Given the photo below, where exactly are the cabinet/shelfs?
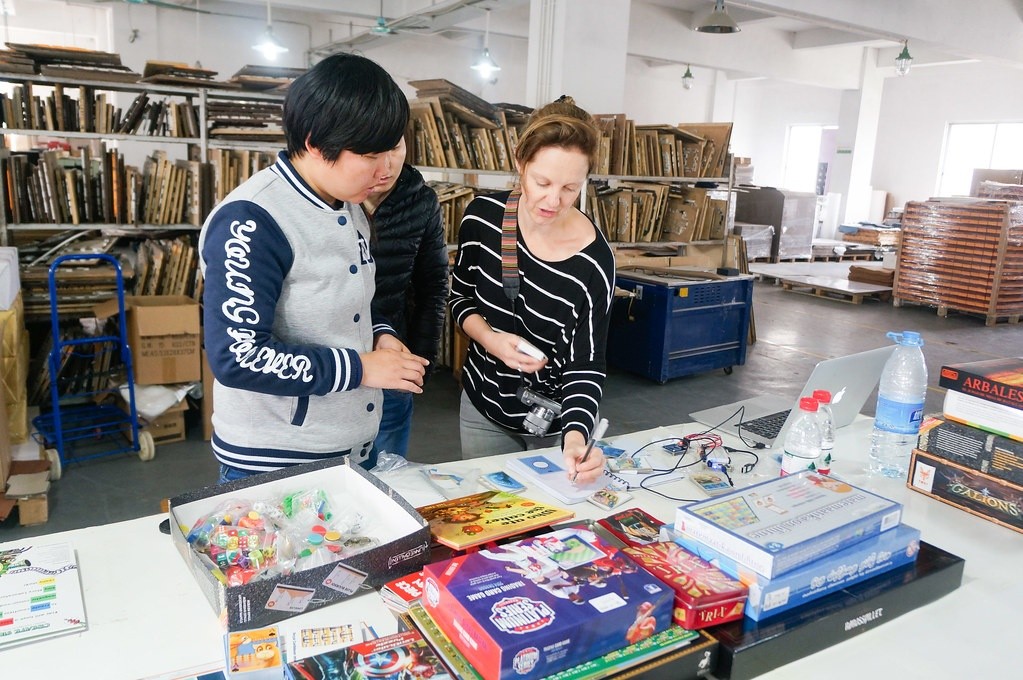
[0,74,735,421]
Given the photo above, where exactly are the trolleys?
[30,252,156,481]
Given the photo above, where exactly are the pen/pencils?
[573,419,611,484]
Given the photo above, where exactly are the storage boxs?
[195,328,216,442]
[166,455,430,631]
[677,469,903,580]
[943,390,1023,440]
[712,544,966,680]
[905,450,1023,533]
[92,294,205,386]
[917,417,1023,486]
[939,357,1023,408]
[660,524,920,622]
[117,396,189,447]
[423,527,676,680]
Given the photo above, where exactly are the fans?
[324,0,430,36]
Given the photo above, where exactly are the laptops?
[688,344,898,448]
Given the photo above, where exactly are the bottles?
[781,397,821,479]
[866,330,928,478]
[811,391,835,476]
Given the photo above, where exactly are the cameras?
[517,386,564,438]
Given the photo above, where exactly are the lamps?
[470,8,501,73]
[252,1,288,58]
[895,40,912,76]
[694,0,741,34]
[682,64,694,89]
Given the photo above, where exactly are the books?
[507,449,629,506]
[907,356,1023,534]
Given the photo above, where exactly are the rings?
[517,364,522,372]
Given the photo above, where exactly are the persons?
[359,131,449,468]
[197,52,430,485]
[446,95,616,484]
